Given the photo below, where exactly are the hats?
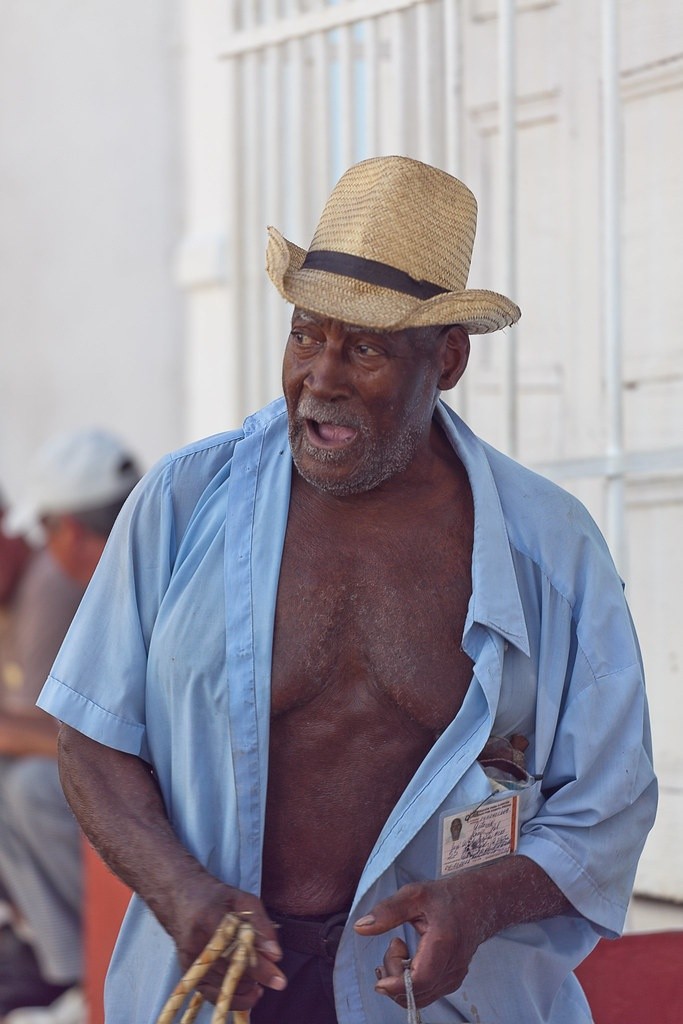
[264,156,522,335]
[2,424,138,537]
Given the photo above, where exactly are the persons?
[0,429,143,1024]
[36,156,659,1024]
[445,818,465,843]
